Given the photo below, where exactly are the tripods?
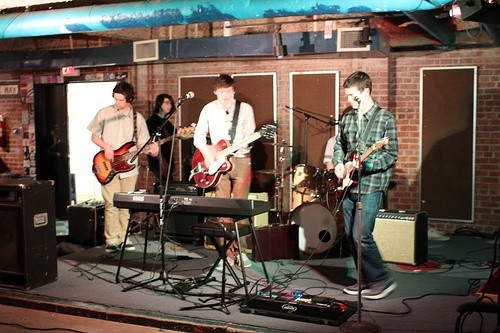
[181,219,251,314]
[123,98,186,300]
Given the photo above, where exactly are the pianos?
[112,191,273,302]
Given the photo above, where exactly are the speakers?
[371,207,428,266]
[0,178,59,290]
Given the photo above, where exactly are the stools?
[192,223,254,294]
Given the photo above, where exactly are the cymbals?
[256,169,293,174]
[263,142,296,147]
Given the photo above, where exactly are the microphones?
[177,91,194,100]
[322,118,335,129]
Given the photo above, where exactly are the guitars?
[93,123,196,185]
[335,137,390,200]
[192,124,277,189]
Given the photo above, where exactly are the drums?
[290,198,347,255]
[321,169,342,200]
[292,164,319,196]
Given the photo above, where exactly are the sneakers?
[343,278,370,295]
[360,277,398,299]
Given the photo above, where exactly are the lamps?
[460,0,500,22]
[270,24,288,58]
[353,15,380,46]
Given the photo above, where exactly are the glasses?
[163,101,172,105]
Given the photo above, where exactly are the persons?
[323,70,398,300]
[88,82,158,254]
[193,74,257,270]
[147,94,194,228]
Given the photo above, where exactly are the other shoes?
[105,243,118,253]
[226,257,234,266]
[119,240,136,251]
[236,253,252,267]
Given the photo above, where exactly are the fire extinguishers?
[0,113,7,148]
